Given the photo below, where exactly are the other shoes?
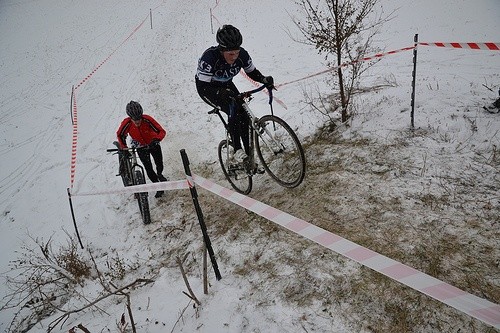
[155,191,164,198]
[159,176,167,182]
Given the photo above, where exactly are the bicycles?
[107,141,151,224]
[208,82,307,195]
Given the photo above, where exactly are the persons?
[117,101,167,198]
[194,24,274,174]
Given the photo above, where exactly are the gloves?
[224,89,235,102]
[153,139,160,146]
[264,76,274,87]
[124,152,133,159]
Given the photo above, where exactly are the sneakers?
[233,150,250,163]
[256,163,265,174]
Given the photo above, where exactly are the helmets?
[126,101,143,120]
[216,24,243,51]
